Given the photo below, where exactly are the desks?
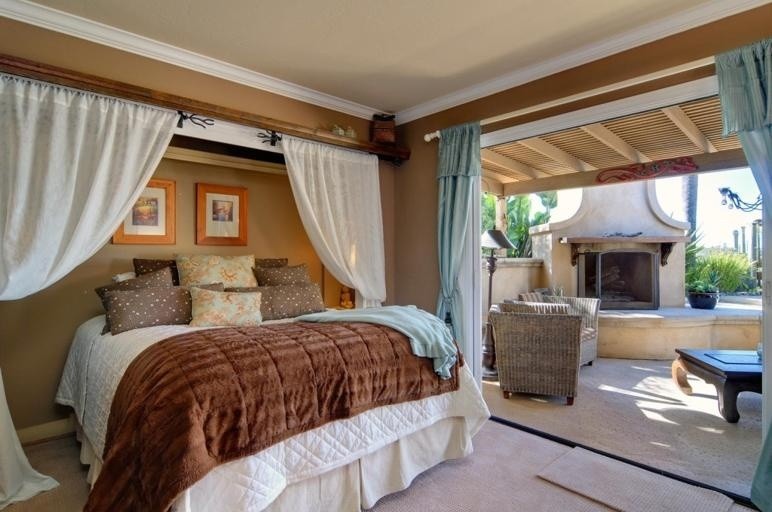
[671,347,763,424]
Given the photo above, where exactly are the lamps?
[479,229,519,383]
[718,185,762,213]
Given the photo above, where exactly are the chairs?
[517,292,601,369]
[488,301,583,408]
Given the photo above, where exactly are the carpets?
[537,442,733,512]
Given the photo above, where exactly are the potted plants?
[685,267,721,311]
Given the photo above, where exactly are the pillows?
[92,256,328,336]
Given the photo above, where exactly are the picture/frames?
[111,178,178,247]
[194,181,249,248]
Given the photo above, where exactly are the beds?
[57,255,491,511]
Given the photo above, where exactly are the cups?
[756,342,762,358]
